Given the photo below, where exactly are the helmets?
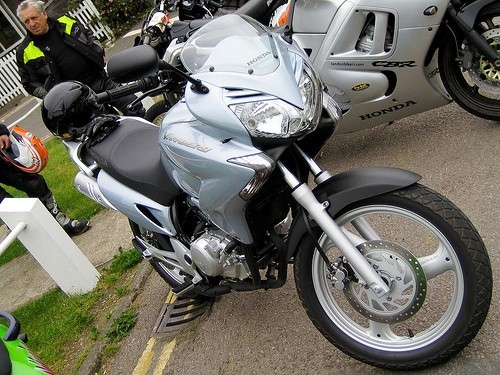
[0,126,48,174]
[41,81,100,140]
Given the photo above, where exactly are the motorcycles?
[131,0,500,127]
[41,0,496,372]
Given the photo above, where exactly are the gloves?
[81,114,119,148]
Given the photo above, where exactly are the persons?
[15,0,147,120]
[0,123,90,238]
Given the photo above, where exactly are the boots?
[40,193,90,237]
